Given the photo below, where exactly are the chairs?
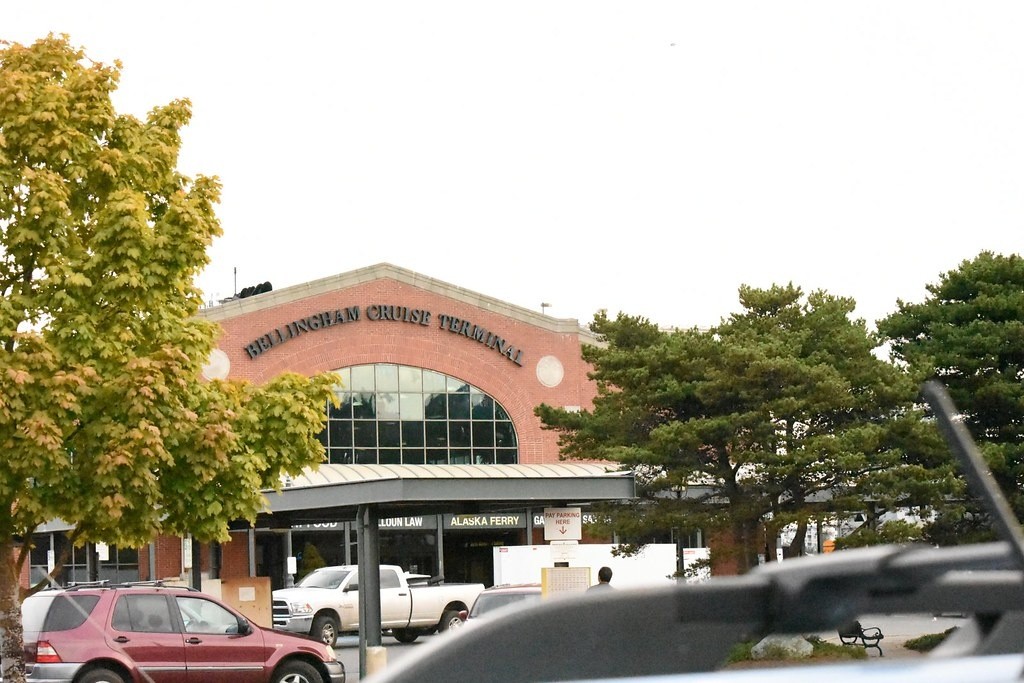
[130,610,168,633]
[837,621,883,657]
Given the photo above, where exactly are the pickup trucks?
[270,564,487,650]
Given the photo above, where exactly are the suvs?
[20,582,346,683]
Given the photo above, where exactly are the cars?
[465,585,545,625]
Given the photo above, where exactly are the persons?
[585,567,617,596]
